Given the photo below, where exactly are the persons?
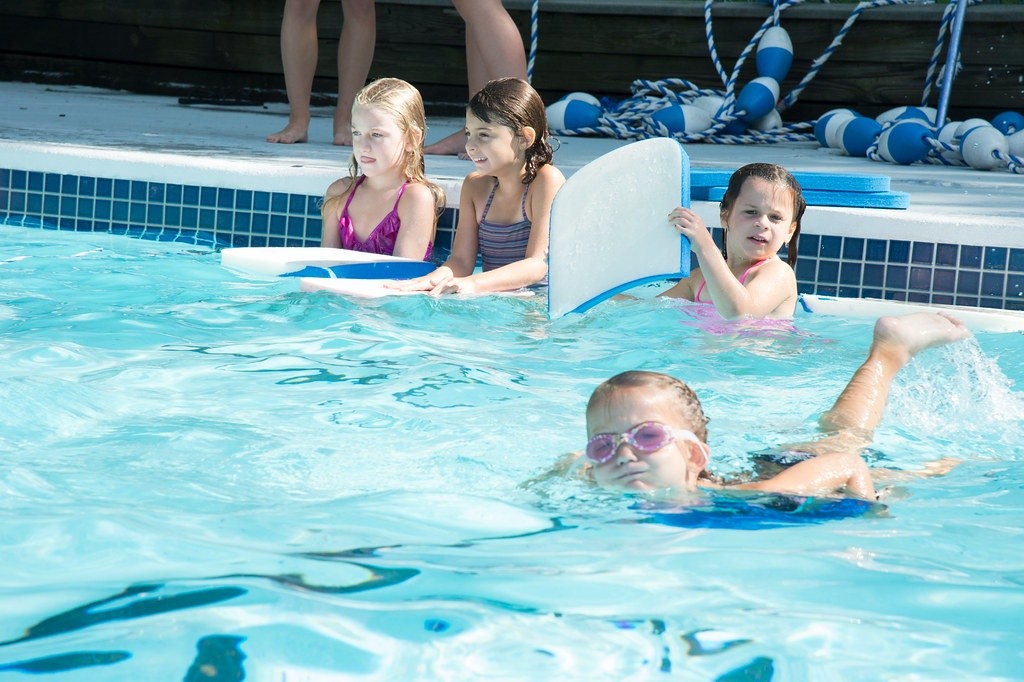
[610,161,806,323]
[265,1,380,146]
[517,310,973,504]
[319,77,438,262]
[416,1,529,163]
[381,77,567,300]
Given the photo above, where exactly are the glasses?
[584,421,675,464]
